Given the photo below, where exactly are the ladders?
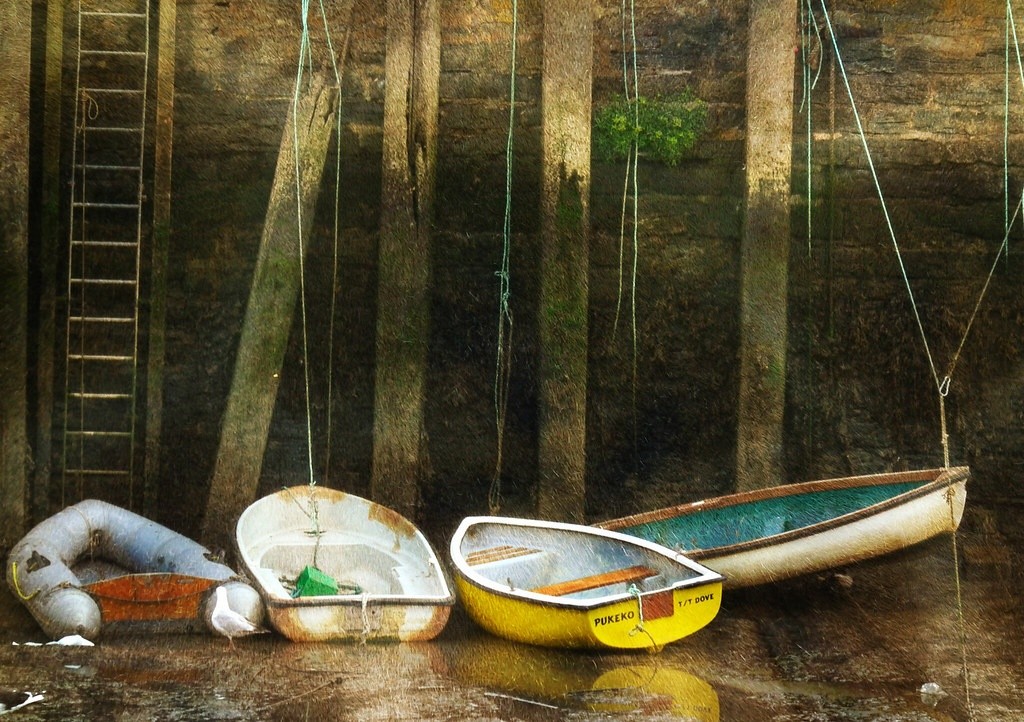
[59,2,152,509]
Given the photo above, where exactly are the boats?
[447,515,729,655]
[7,497,264,645]
[232,485,457,644]
[582,465,970,592]
